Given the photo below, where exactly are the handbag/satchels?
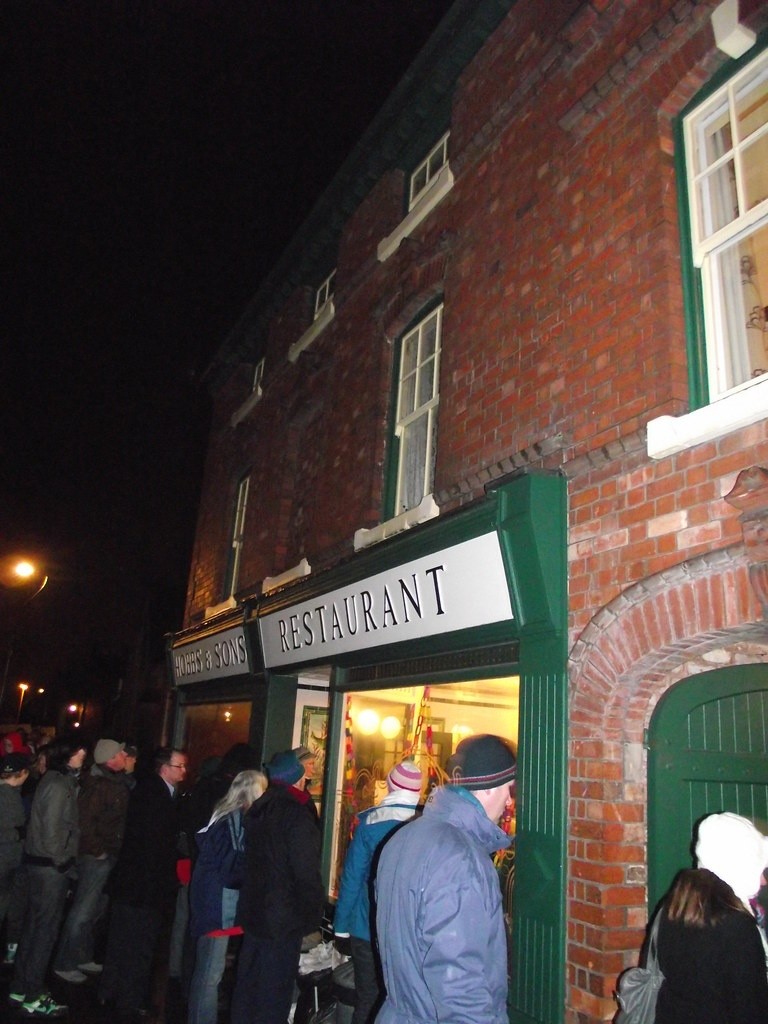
[613,908,664,1024]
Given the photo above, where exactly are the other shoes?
[55,971,87,983]
[77,959,103,972]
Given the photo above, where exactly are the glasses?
[167,763,185,768]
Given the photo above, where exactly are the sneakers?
[8,991,50,1006]
[19,993,67,1018]
[4,944,18,963]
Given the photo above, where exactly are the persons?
[639,810,768,1024]
[1,726,140,1018]
[114,748,194,1021]
[172,742,325,1024]
[333,735,519,1024]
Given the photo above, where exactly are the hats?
[444,734,516,790]
[266,750,305,785]
[695,810,768,904]
[387,762,422,793]
[294,747,315,762]
[0,752,33,773]
[94,739,125,763]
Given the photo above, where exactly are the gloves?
[334,936,352,957]
